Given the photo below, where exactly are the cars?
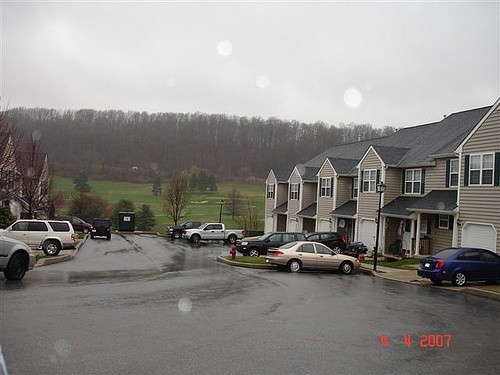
[265,242,361,275]
[0,235,35,280]
[416,248,500,287]
[236,231,348,257]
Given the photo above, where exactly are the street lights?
[218,200,224,223]
[373,182,388,269]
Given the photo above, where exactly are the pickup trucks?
[182,223,244,245]
[167,221,221,239]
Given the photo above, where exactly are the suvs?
[91,218,111,240]
[50,217,92,234]
[0,219,77,256]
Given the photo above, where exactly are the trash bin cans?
[118,211,136,232]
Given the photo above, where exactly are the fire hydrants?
[230,246,237,260]
